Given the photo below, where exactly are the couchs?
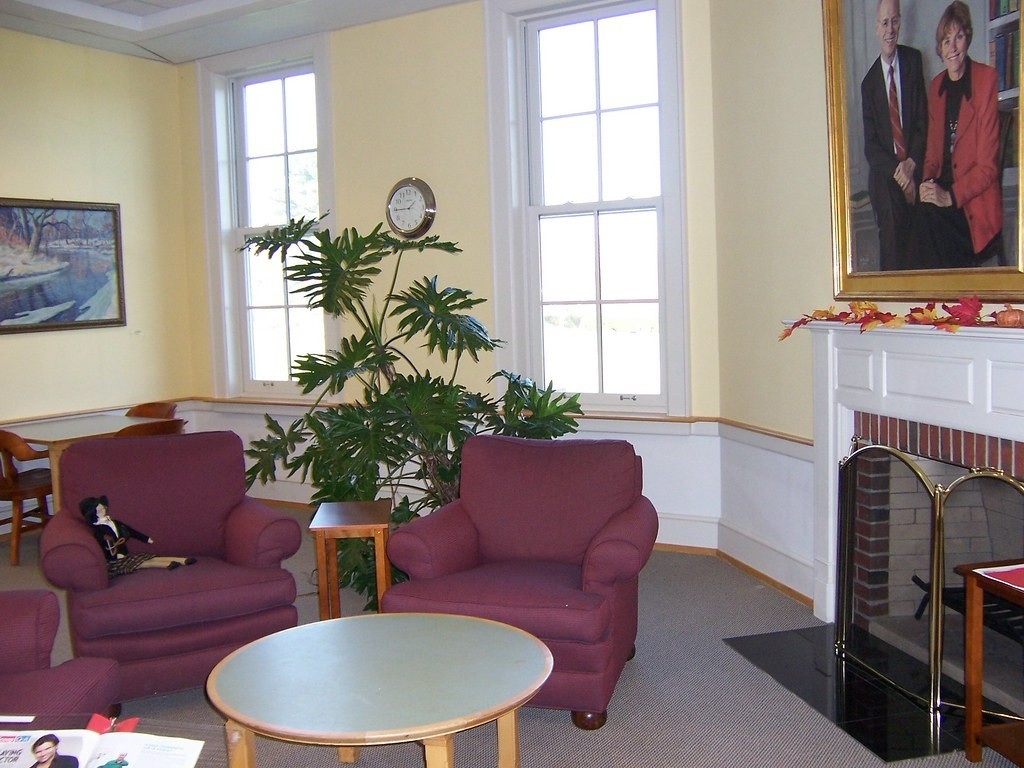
[381,434,659,729]
[40,428,302,718]
[0,589,123,729]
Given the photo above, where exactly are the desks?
[0,415,155,514]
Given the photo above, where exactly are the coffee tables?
[206,611,554,768]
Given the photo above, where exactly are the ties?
[888,66,906,160]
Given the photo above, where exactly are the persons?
[79,495,198,576]
[97,752,128,768]
[29,734,79,768]
[907,0,1002,269]
[860,0,926,272]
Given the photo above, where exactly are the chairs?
[0,429,53,566]
[125,401,177,419]
[113,419,184,436]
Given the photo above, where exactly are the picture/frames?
[821,0,1024,303]
[0,197,127,335]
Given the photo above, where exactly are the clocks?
[386,177,437,239]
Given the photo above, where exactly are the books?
[989,29,1019,92]
[998,107,1018,167]
[989,0,1021,21]
[0,730,205,768]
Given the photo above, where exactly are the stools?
[309,499,391,622]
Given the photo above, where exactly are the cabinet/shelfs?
[953,557,1024,768]
[984,0,1019,187]
